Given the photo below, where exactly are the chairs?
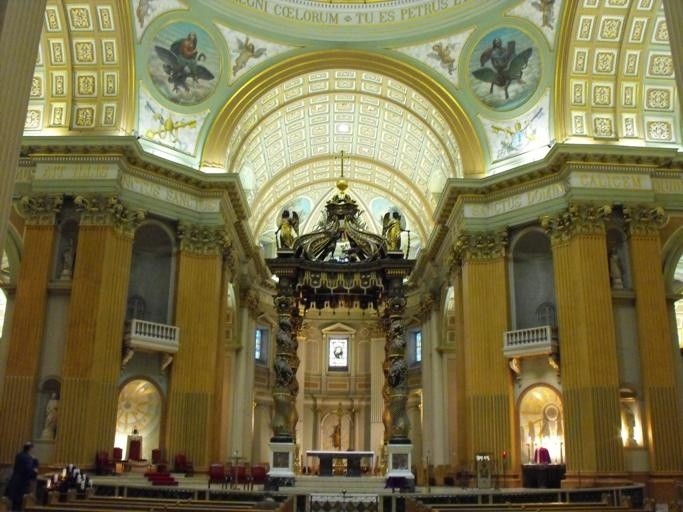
[96,433,269,489]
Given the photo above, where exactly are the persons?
[39,391,59,438]
[56,236,76,283]
[177,31,200,87]
[0,440,92,512]
[382,211,409,251]
[489,37,524,84]
[230,43,266,78]
[608,244,624,291]
[431,44,456,76]
[274,210,295,250]
